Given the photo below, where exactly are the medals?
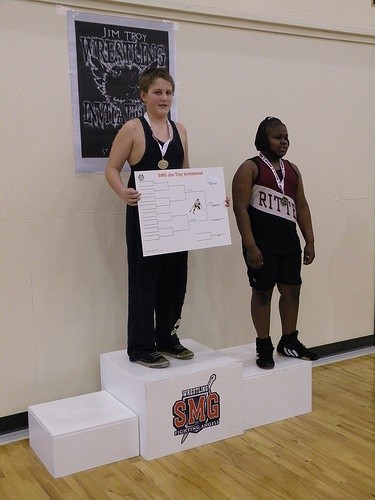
[158,160,168,170]
[281,197,288,206]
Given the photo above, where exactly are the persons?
[106,68,230,369]
[232,116,319,369]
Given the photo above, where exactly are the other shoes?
[156,342,194,360]
[133,351,170,369]
[276,331,319,361]
[255,336,275,370]
